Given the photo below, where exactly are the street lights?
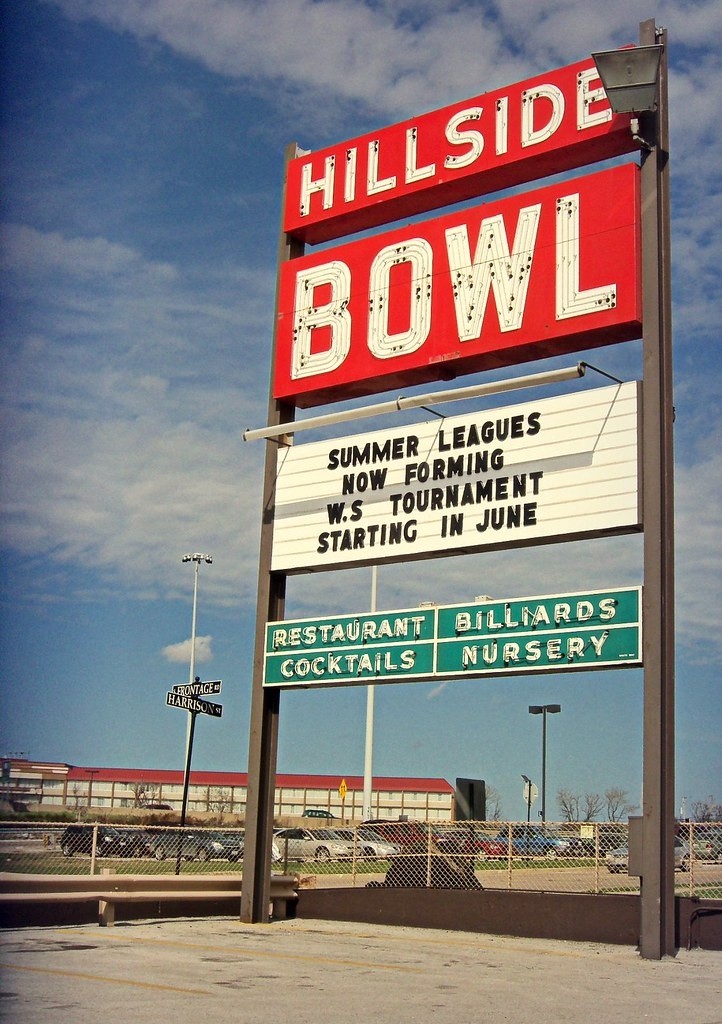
[181,552,212,819]
[529,704,561,831]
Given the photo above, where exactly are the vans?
[605,834,693,874]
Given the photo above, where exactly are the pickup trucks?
[496,825,593,861]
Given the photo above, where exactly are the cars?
[435,828,514,862]
[690,831,722,862]
[273,828,363,864]
[114,829,152,858]
[328,829,403,860]
[356,819,392,841]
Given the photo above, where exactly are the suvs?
[144,822,245,862]
[302,810,341,819]
[372,819,440,848]
[59,823,134,857]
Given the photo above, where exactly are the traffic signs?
[165,680,222,717]
